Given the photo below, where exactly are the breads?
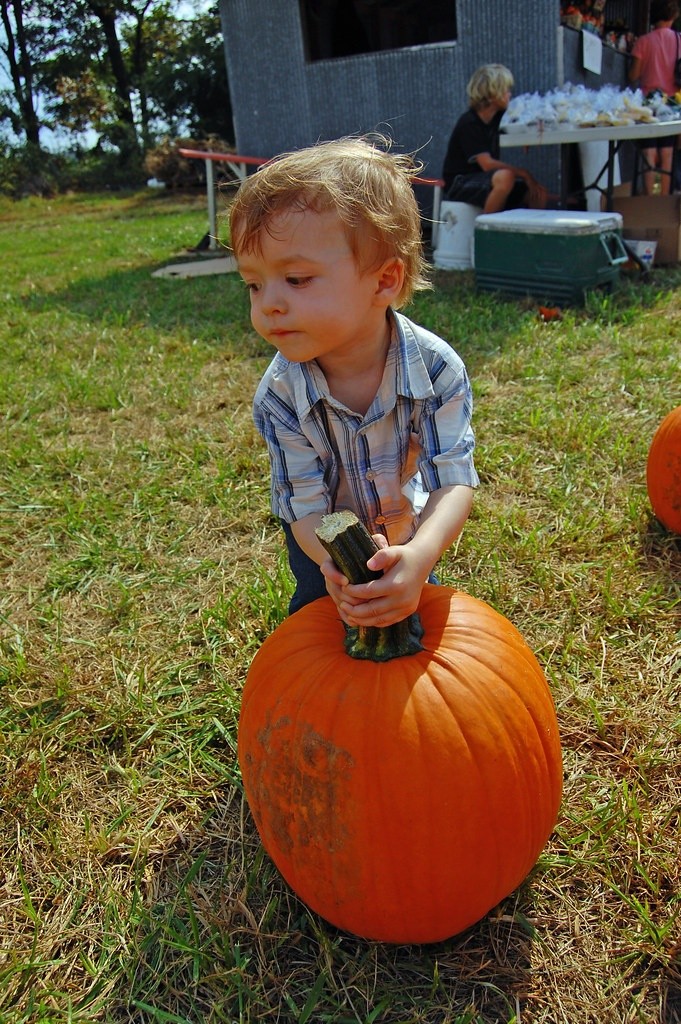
[573,105,654,126]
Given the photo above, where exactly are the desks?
[495,121,681,210]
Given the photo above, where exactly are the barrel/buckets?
[432,201,484,270]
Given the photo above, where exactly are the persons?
[627,0,681,195]
[442,63,549,214]
[226,136,481,629]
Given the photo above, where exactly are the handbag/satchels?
[674,31,681,88]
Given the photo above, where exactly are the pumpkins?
[647,404,681,538]
[238,510,563,945]
[538,305,558,321]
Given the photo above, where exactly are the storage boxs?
[599,182,681,268]
[476,207,629,312]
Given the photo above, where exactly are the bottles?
[619,35,626,51]
